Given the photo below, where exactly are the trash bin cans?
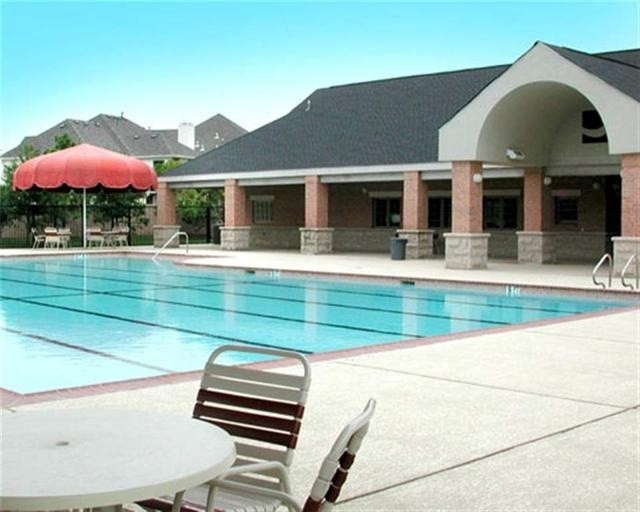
[213,219,224,244]
[390,236,407,260]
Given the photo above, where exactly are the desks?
[0,404,236,512]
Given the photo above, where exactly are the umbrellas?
[12,143,158,248]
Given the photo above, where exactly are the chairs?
[115,344,377,510]
[31,223,131,250]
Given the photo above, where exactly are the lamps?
[506,148,525,161]
